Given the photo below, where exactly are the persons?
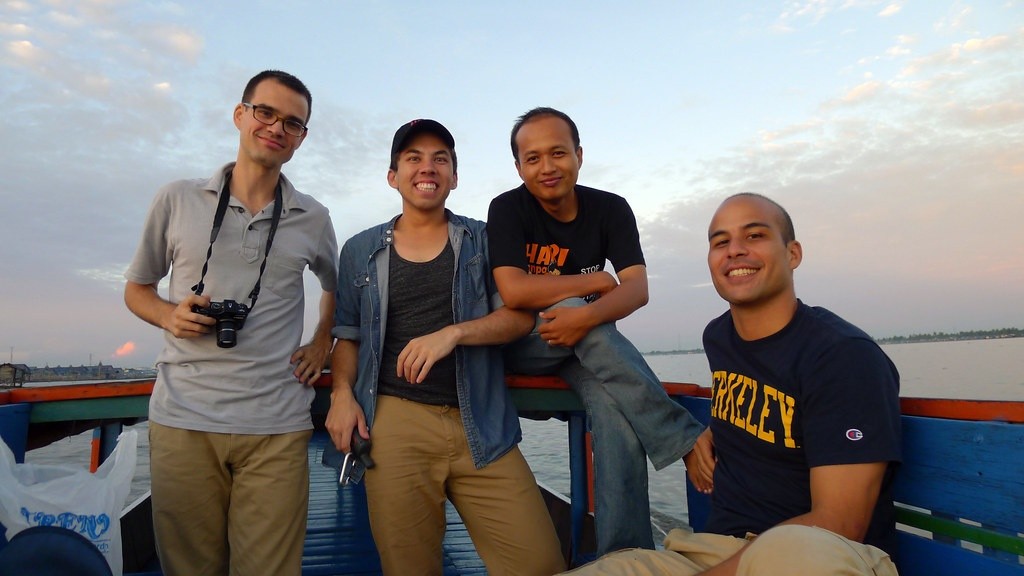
[326,118,568,575]
[125,71,339,576]
[486,108,712,560]
[555,194,898,575]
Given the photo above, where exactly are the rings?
[549,340,552,346]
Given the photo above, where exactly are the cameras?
[190,300,250,349]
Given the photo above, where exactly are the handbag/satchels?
[0,431,138,576]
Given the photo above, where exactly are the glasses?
[243,103,308,137]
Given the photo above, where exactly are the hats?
[392,118,455,154]
